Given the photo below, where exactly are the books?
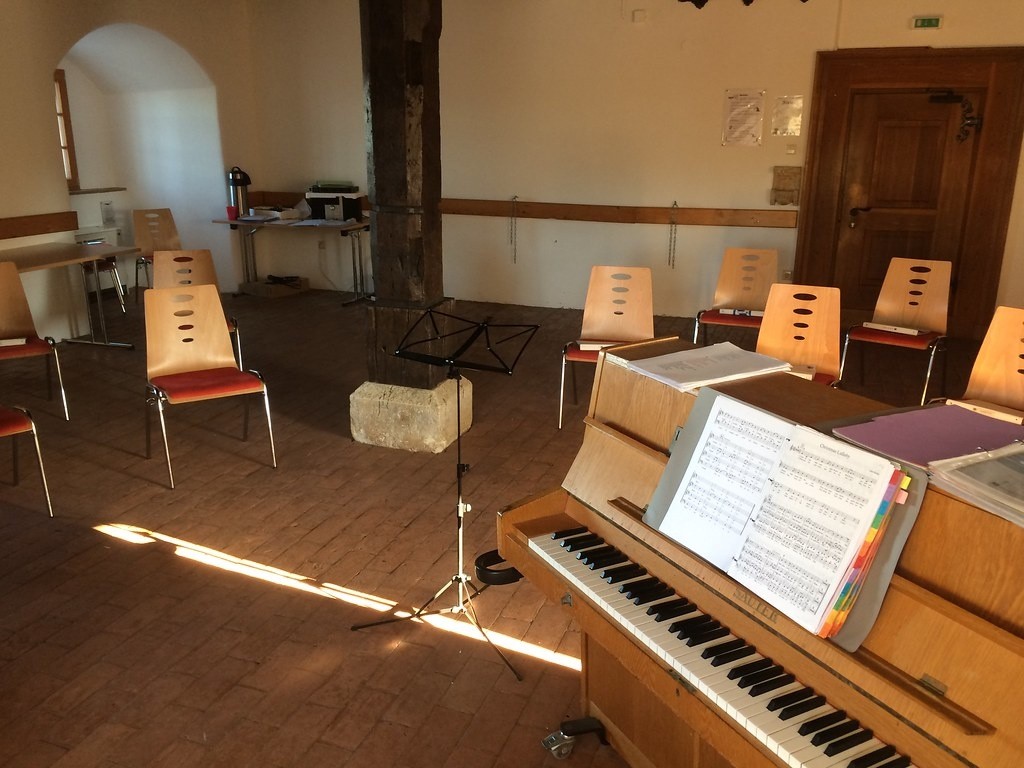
[641,386,928,653]
[831,404,1024,528]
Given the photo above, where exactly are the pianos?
[494,332,1024,768]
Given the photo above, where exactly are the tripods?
[352,304,540,680]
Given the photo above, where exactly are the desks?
[213,217,372,304]
[0,242,141,349]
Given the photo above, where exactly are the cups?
[225,206,235,222]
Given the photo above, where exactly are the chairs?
[692,249,777,348]
[927,306,1024,422]
[0,404,53,518]
[133,207,183,302]
[839,255,952,407]
[558,264,654,431]
[142,285,277,490]
[154,250,244,373]
[755,284,842,390]
[0,260,71,422]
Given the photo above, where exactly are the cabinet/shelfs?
[76,225,130,293]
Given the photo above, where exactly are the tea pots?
[230,166,252,217]
